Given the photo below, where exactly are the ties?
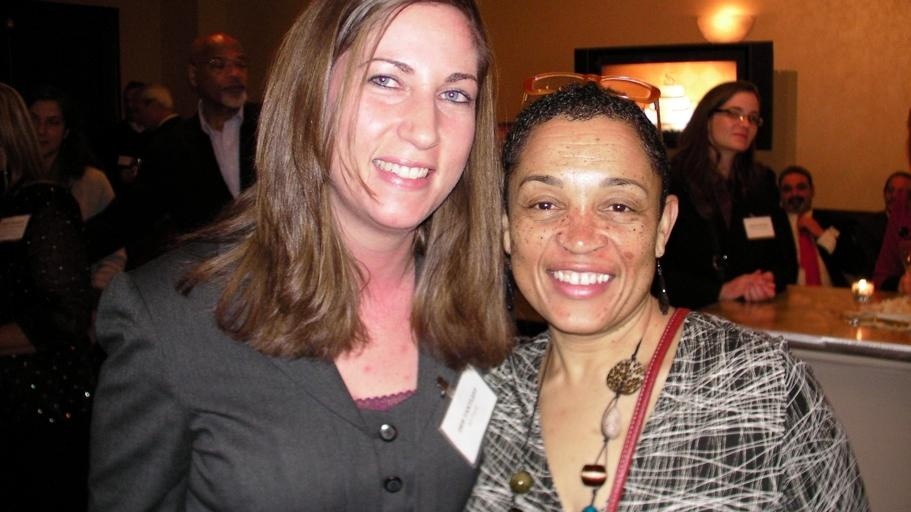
[796,213,823,285]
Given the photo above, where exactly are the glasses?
[515,70,665,147]
[716,107,765,128]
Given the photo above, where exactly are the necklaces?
[510,340,649,511]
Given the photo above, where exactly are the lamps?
[691,13,753,43]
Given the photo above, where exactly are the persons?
[1,27,264,512]
[650,79,801,311]
[857,170,911,285]
[83,2,486,511]
[773,164,857,289]
[457,77,873,511]
[871,187,911,296]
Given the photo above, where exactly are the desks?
[693,284,911,511]
